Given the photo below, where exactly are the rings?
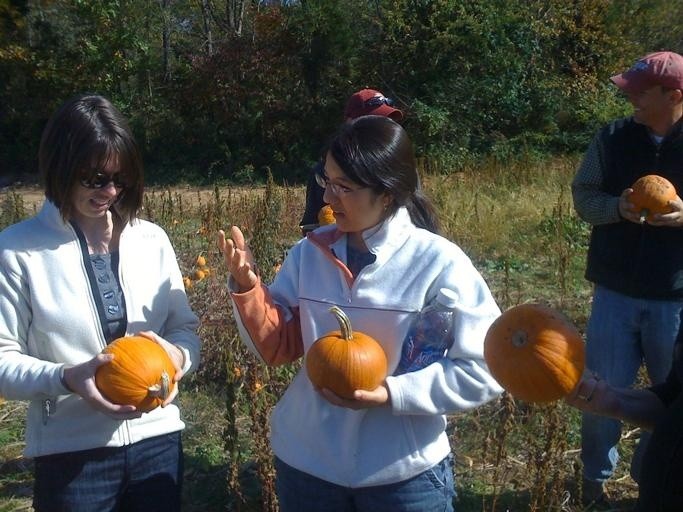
[576,393,591,402]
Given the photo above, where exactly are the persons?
[569,52,682,509]
[0,93,203,512]
[215,113,507,512]
[563,310,683,511]
[299,87,420,238]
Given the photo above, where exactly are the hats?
[343,88,402,123]
[609,51,683,94]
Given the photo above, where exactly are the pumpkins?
[183,255,210,287]
[305,306,388,400]
[95,335,176,412]
[318,204,336,226]
[627,174,676,225]
[483,304,584,402]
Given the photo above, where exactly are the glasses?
[313,169,369,196]
[364,95,393,110]
[629,57,649,78]
[77,166,135,190]
